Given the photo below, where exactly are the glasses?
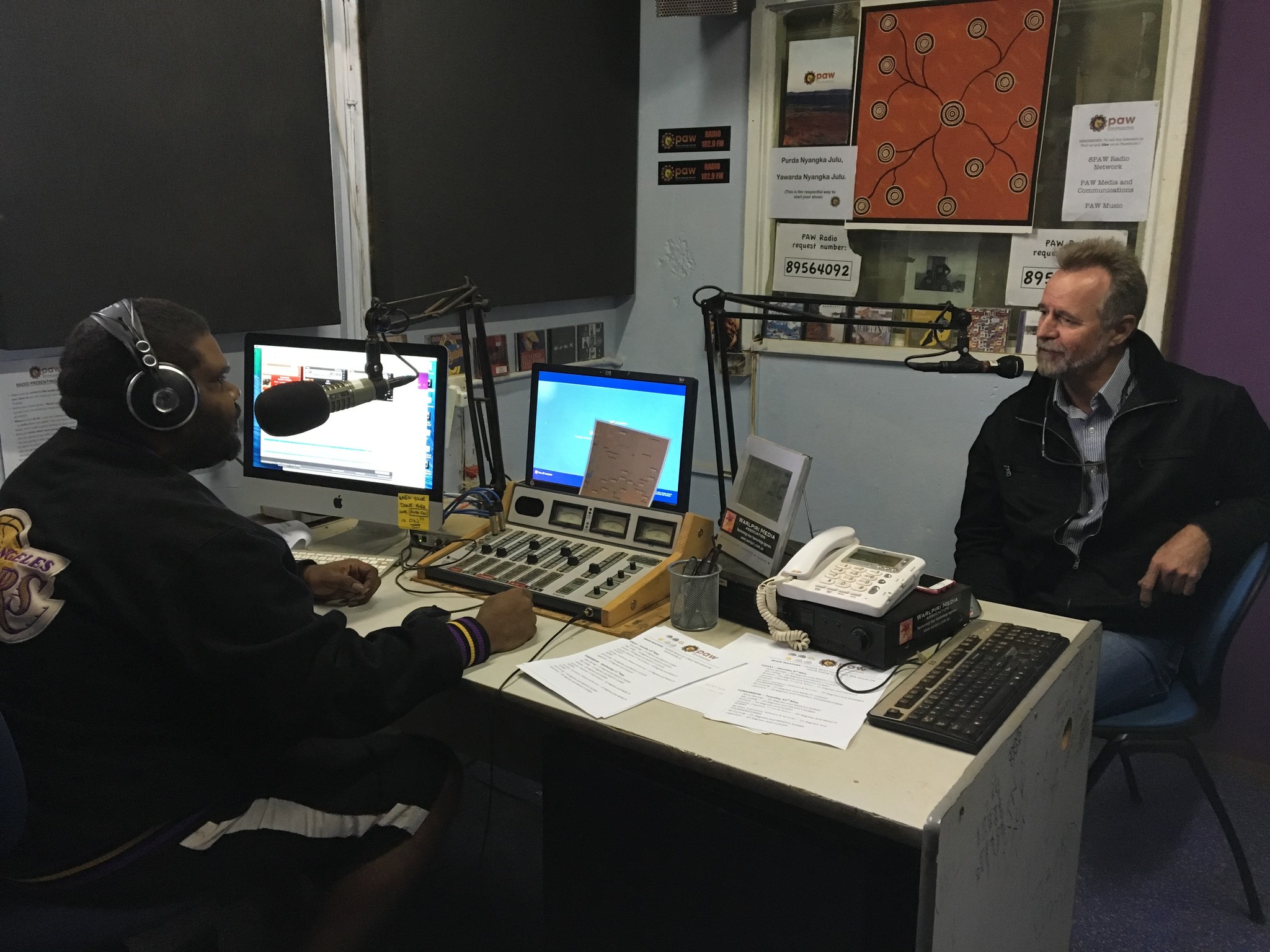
[1042,395,1124,466]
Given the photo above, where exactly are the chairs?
[1084,541,1270,926]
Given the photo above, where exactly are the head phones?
[90,298,198,433]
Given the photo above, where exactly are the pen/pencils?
[701,544,722,589]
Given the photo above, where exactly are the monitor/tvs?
[243,333,451,559]
[524,362,700,515]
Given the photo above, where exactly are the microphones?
[917,354,1023,379]
[254,376,418,438]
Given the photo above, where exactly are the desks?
[249,511,1102,952]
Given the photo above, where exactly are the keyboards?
[290,548,400,578]
[866,620,1071,758]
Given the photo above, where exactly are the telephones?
[775,526,926,617]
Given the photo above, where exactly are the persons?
[0,297,536,952]
[953,237,1270,721]
